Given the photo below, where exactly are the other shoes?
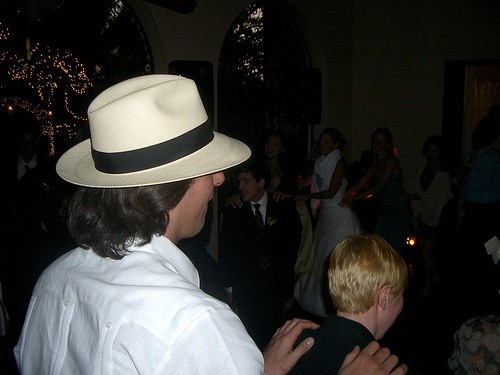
[280,297,297,317]
[418,287,430,303]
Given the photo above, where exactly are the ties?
[253,204,264,228]
[24,165,36,176]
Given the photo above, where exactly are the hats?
[56,74,252,189]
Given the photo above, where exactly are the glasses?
[20,140,34,145]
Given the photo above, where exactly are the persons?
[337,114,500,285]
[286,233,409,375]
[12,73,410,374]
[291,126,365,319]
[219,129,300,322]
